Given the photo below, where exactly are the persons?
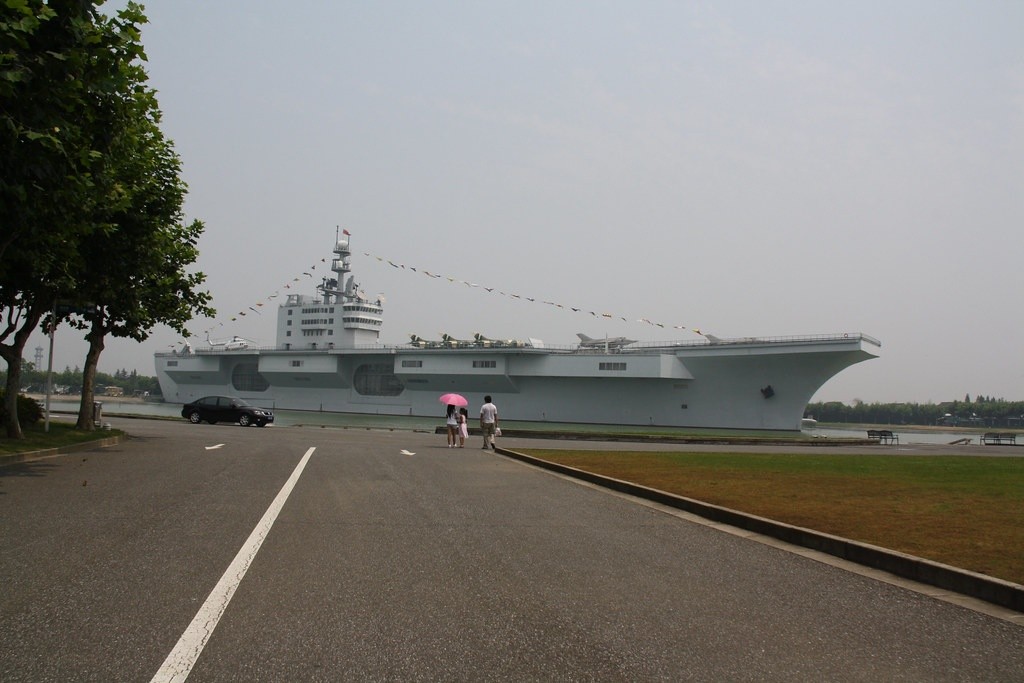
[480,395,499,450]
[445,403,460,448]
[457,408,469,448]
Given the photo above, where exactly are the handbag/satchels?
[494,426,502,437]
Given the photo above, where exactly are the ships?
[154,223,882,433]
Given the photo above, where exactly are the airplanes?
[576,332,637,348]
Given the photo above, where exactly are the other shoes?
[453,444,457,448]
[491,443,495,449]
[459,444,463,448]
[448,444,451,447]
[482,446,488,449]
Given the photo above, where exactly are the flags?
[343,228,349,235]
[169,259,327,348]
[364,252,704,336]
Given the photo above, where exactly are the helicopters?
[206,331,260,350]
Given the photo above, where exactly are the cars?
[181,395,274,427]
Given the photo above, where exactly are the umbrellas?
[439,393,468,406]
